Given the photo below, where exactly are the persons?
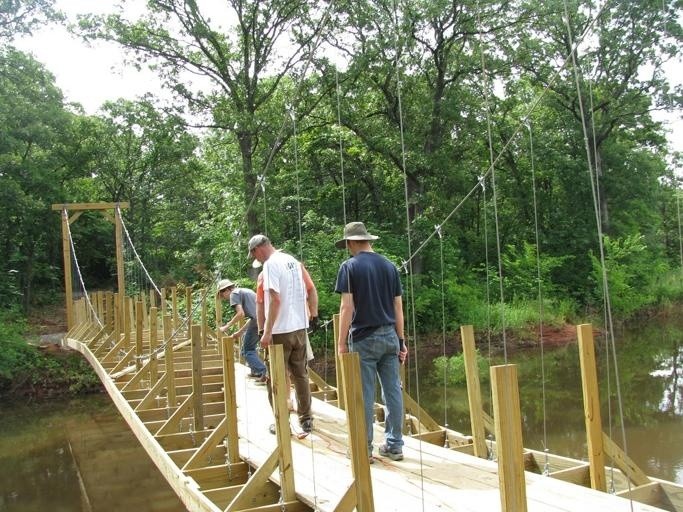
[334,221,408,461]
[252,248,314,411]
[217,279,268,386]
[247,235,318,437]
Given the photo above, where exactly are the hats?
[335,222,378,249]
[247,235,268,259]
[217,279,235,300]
[252,249,284,268]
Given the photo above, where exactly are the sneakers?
[346,448,374,464]
[270,424,293,436]
[299,420,313,431]
[248,374,269,384]
[379,444,403,461]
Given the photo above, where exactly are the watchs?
[258,329,264,334]
